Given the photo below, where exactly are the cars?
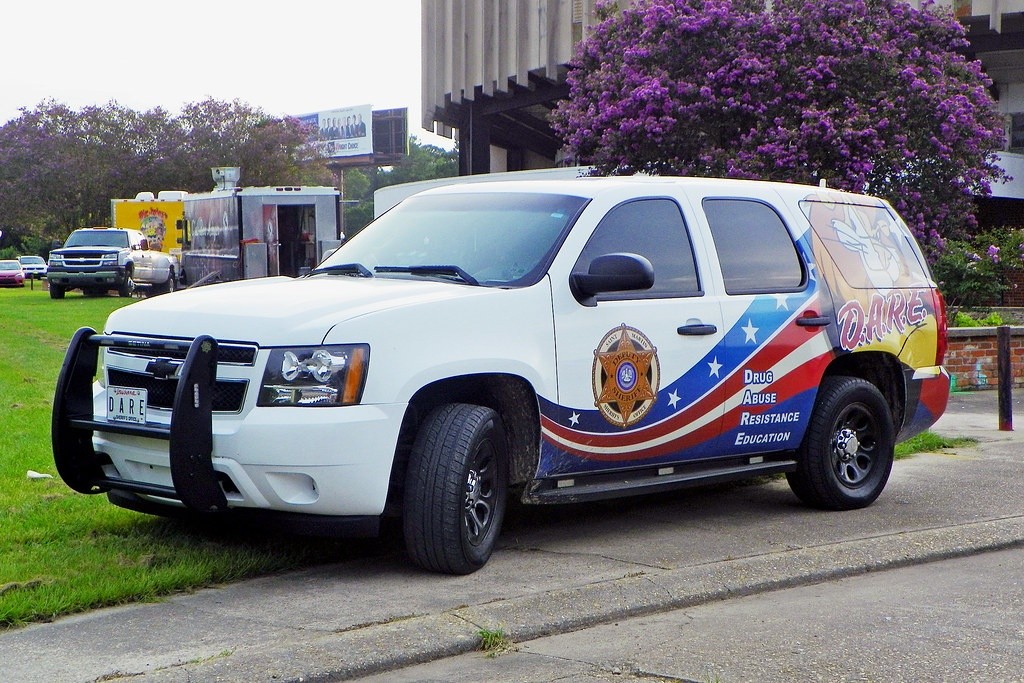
[0,259,25,287]
[17,255,48,280]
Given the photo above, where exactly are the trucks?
[175,166,343,289]
[111,189,191,286]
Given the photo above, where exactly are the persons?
[319,114,366,141]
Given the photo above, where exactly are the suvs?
[48,174,950,576]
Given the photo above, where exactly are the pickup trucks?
[46,226,180,299]
